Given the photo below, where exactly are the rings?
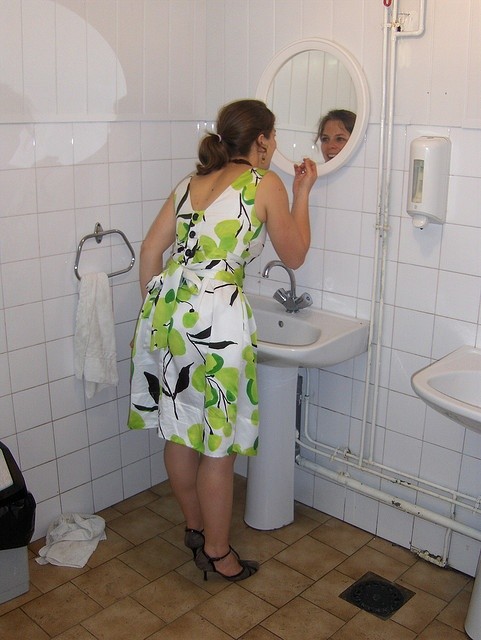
[302,168,307,173]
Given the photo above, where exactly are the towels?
[71,271,123,403]
[37,513,106,572]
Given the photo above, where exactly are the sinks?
[243,293,371,368]
[411,343,480,437]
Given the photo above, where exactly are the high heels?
[195,544,261,583]
[185,527,205,561]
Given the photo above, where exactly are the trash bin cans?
[0,442,37,603]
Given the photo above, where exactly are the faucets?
[262,258,313,314]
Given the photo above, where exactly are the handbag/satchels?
[1,441,37,551]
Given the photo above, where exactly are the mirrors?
[246,34,371,185]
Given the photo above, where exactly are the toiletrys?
[406,135,453,230]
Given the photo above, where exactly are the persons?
[316,109,357,162]
[128,99,318,582]
